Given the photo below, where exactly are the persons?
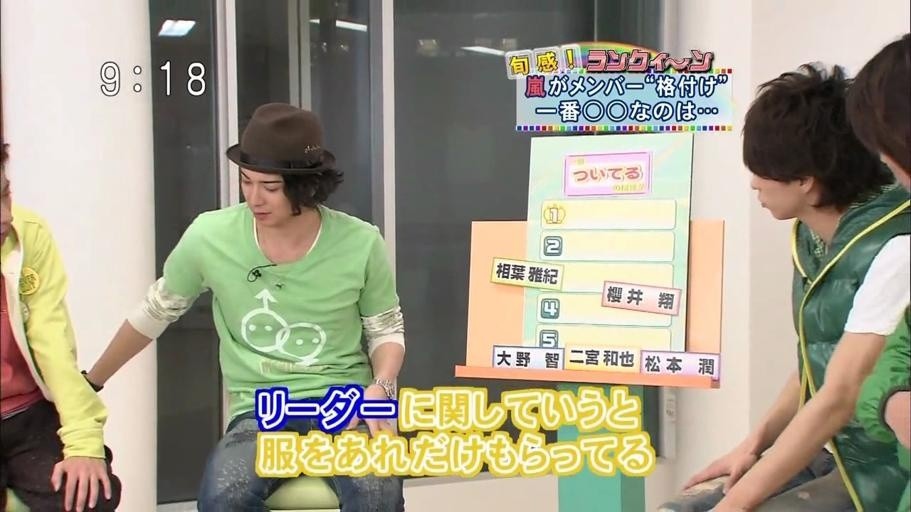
[82,102,405,512]
[0,144,121,512]
[846,34,911,512]
[656,61,911,511]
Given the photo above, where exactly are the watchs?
[372,377,399,401]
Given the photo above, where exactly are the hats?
[223,102,337,176]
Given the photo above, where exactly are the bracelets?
[82,369,104,394]
